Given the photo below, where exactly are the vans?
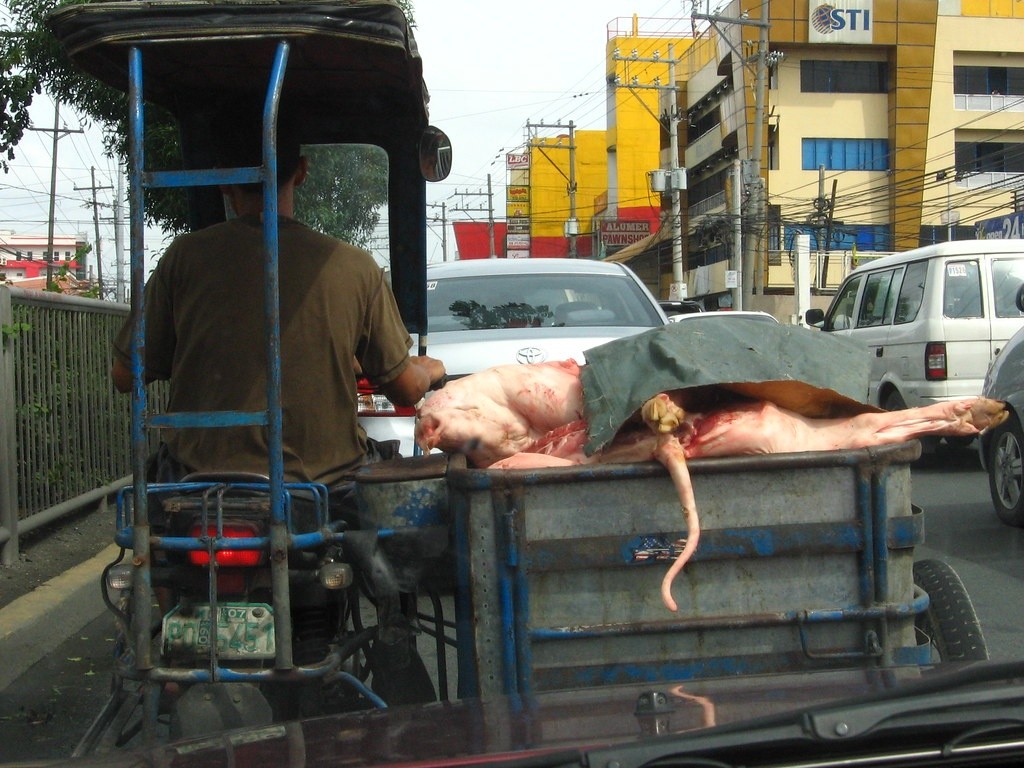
[805,239,1024,455]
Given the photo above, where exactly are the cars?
[658,299,781,325]
[978,281,1024,528]
[356,257,674,458]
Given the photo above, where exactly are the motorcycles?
[41,0,992,757]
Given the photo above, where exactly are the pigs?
[414,317,1008,611]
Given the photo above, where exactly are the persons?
[114,135,444,704]
